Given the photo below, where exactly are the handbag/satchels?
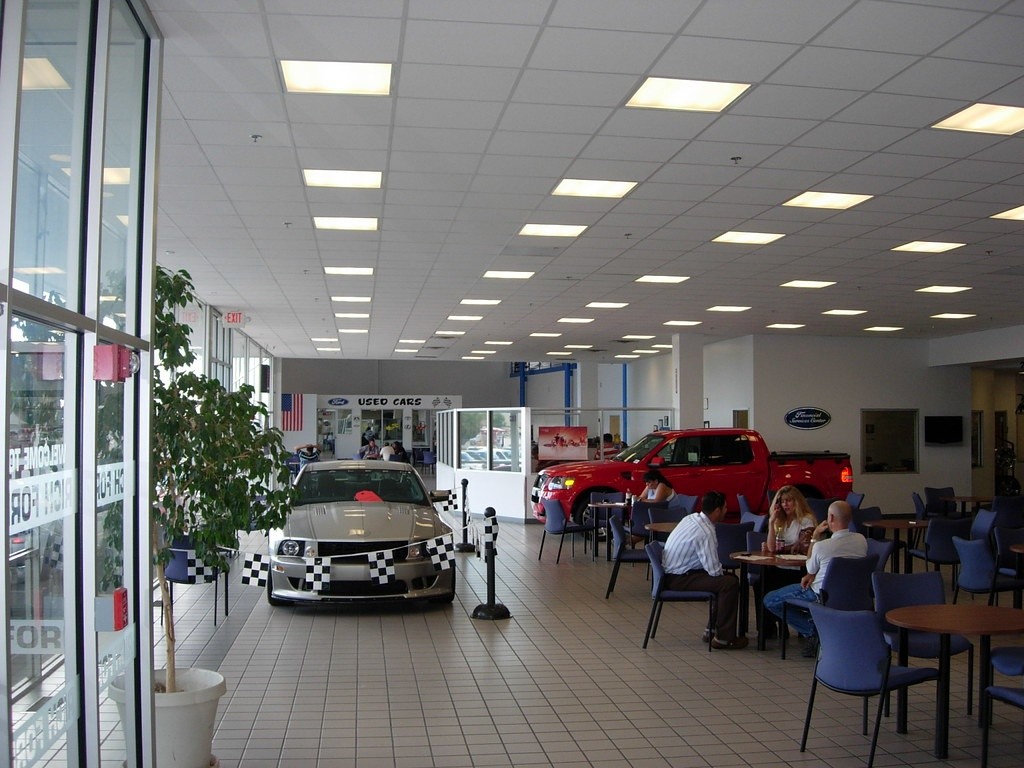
[799,527,826,555]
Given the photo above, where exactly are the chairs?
[160,534,228,626]
[378,479,405,497]
[283,454,301,479]
[358,447,436,476]
[314,475,341,501]
[538,486,1024,768]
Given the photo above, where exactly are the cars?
[267,459,456,606]
[460,446,512,464]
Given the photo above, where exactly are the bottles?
[775,527,785,554]
[625,488,632,505]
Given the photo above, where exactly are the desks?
[729,552,807,650]
[588,502,630,561]
[1010,544,1024,553]
[885,604,1024,759]
[288,461,301,477]
[942,496,996,519]
[644,523,678,533]
[862,520,930,573]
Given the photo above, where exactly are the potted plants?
[105,265,301,768]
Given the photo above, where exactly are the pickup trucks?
[530,427,853,542]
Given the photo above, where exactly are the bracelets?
[810,539,817,543]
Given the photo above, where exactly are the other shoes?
[702,629,714,641]
[712,635,749,650]
[802,635,819,657]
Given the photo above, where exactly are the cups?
[790,544,800,554]
[631,495,637,506]
[761,542,768,554]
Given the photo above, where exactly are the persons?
[595,434,619,460]
[763,500,869,656]
[661,490,749,650]
[380,442,395,461]
[611,469,678,546]
[327,432,333,443]
[357,438,379,459]
[295,443,322,461]
[362,426,375,446]
[753,485,818,641]
[392,441,406,456]
